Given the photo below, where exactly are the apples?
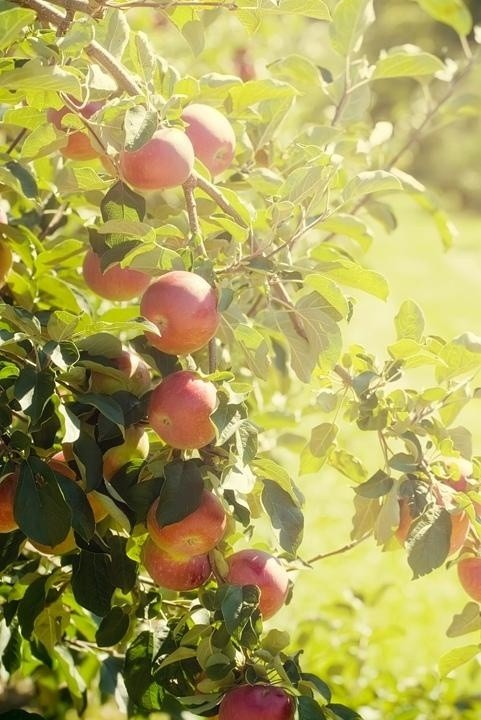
[1,84,480,720]
